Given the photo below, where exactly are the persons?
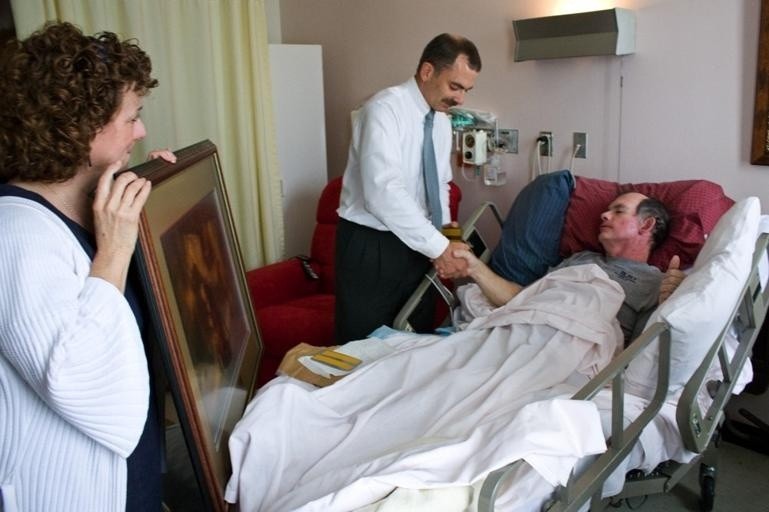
[335,33,481,346]
[1,20,180,512]
[434,192,688,354]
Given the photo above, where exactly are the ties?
[424,108,441,229]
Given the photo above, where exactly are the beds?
[225,179,769,512]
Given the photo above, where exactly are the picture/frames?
[113,138,264,512]
[750,1,769,166]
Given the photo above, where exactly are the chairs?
[244,177,462,373]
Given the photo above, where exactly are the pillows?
[490,171,573,286]
[622,195,761,398]
[561,178,733,265]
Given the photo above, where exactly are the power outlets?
[539,132,552,157]
[572,131,586,158]
